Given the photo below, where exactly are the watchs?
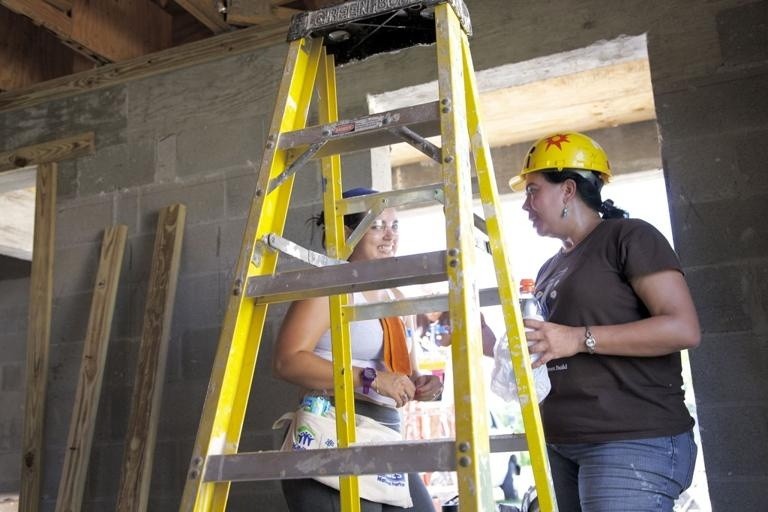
[357,366,380,395]
[583,325,597,357]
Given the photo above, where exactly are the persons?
[481,130,702,512]
[274,188,445,511]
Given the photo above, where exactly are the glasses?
[347,218,406,236]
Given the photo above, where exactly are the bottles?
[302,386,331,415]
[516,276,543,326]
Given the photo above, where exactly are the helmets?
[506,128,614,193]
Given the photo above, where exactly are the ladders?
[179,0,560,512]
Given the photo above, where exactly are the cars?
[487,406,523,502]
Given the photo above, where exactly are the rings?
[432,393,436,400]
